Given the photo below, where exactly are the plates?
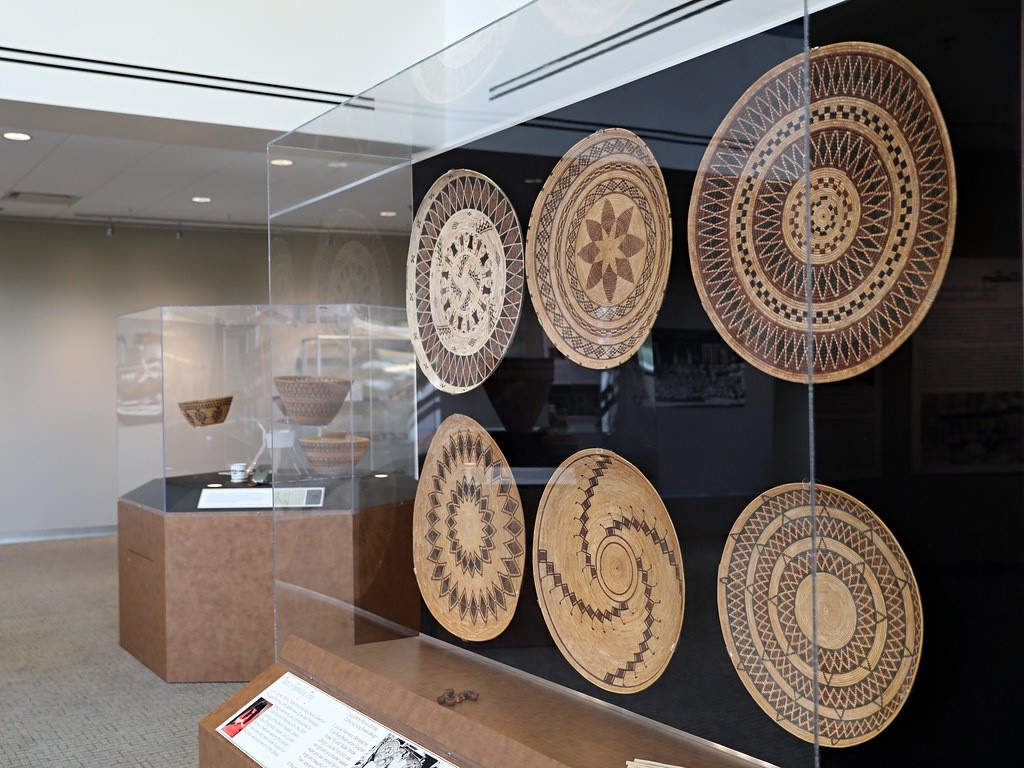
[714,482,925,748]
[411,412,526,643]
[687,41,958,386]
[402,166,526,396]
[530,447,685,694]
[525,127,675,370]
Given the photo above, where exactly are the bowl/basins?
[274,375,354,426]
[176,395,233,429]
[299,433,372,477]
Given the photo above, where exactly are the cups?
[229,462,248,483]
